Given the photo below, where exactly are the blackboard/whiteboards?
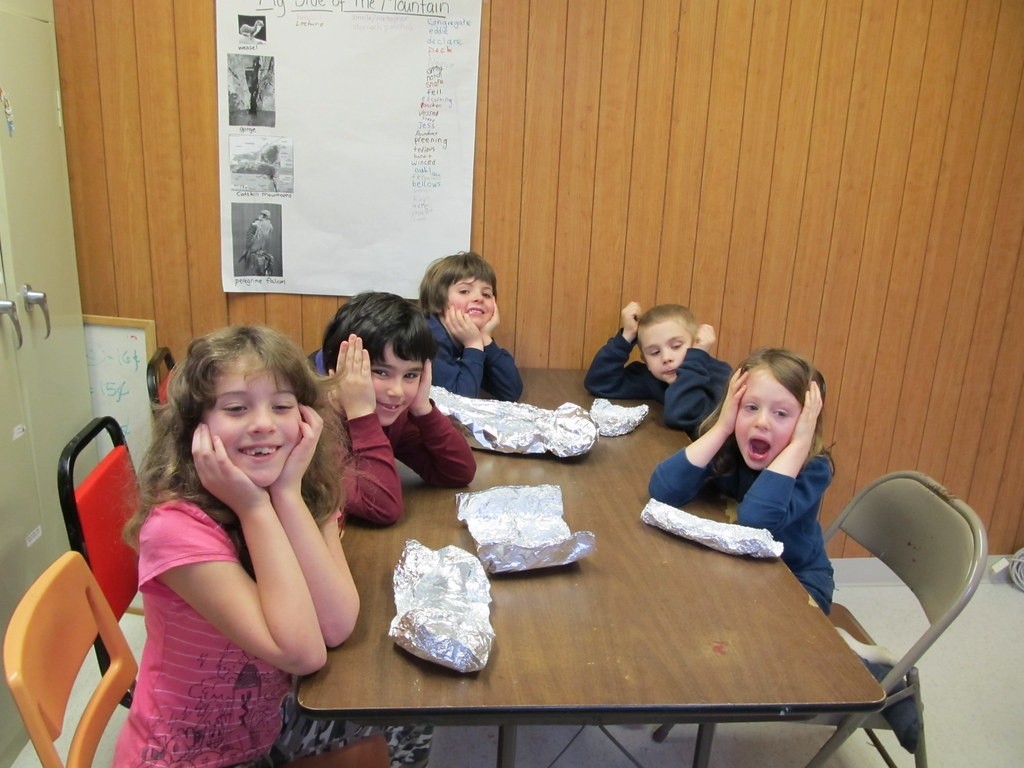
[83,314,158,473]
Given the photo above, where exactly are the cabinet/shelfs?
[0,0,104,768]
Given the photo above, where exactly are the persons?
[649,348,920,753]
[418,251,523,402]
[113,324,434,768]
[584,301,735,441]
[308,292,477,526]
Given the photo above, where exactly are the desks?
[296,364,886,768]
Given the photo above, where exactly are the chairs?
[651,469,987,768]
[57,414,142,710]
[146,345,176,415]
[4,548,392,768]
